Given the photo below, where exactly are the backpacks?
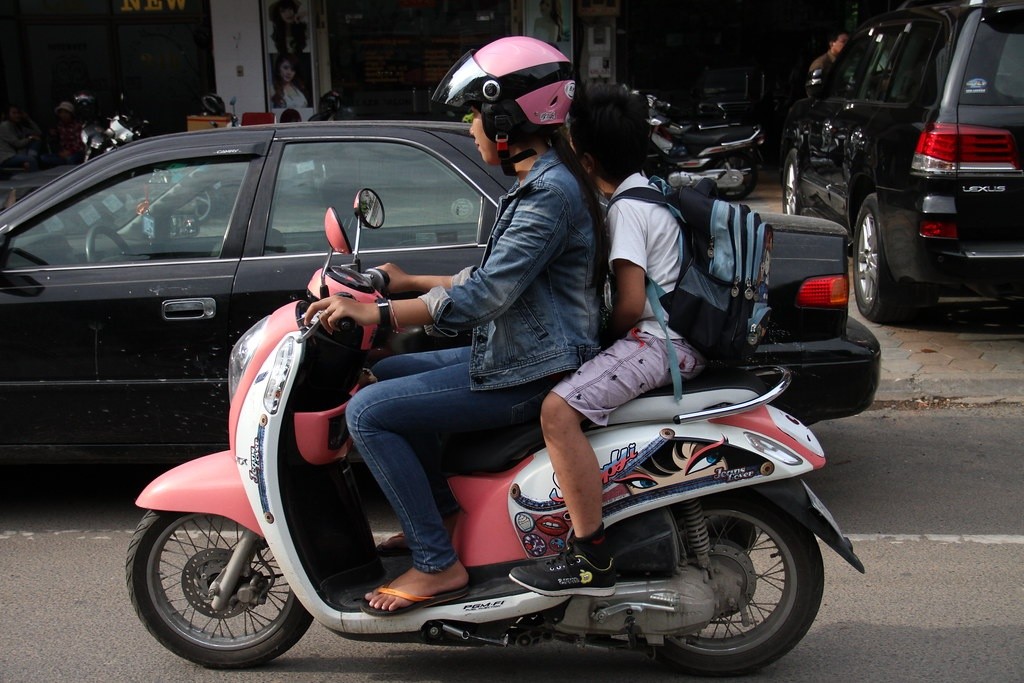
[605,178,773,366]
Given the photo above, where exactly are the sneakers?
[509,544,618,596]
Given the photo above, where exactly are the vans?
[777,0,1024,323]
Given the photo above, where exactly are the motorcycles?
[619,85,765,201]
[56,89,356,235]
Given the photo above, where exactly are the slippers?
[360,581,468,617]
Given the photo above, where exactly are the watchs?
[374,296,394,336]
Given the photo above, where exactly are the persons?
[802,29,849,97]
[267,0,308,52]
[268,53,308,109]
[507,87,708,600]
[304,37,608,619]
[0,101,86,175]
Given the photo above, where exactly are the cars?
[0,121,880,475]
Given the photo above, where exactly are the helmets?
[447,35,574,145]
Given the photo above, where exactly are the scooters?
[126,188,866,679]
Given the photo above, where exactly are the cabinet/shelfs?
[188,114,232,132]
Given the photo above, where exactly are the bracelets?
[28,135,34,140]
[387,300,401,336]
